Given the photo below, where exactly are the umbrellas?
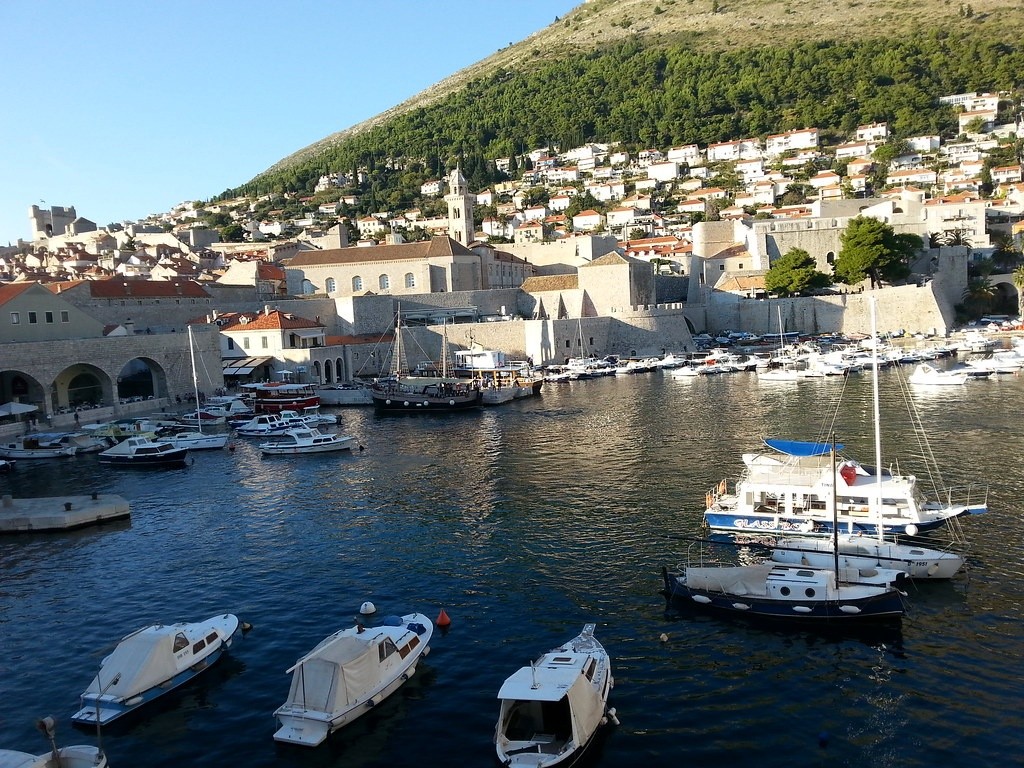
[0,401,38,417]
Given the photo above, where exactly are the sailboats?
[350,300,483,413]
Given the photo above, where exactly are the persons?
[146,327,152,335]
[73,412,84,429]
[484,374,503,390]
[445,382,461,394]
[171,328,176,331]
[215,377,290,397]
[401,384,421,394]
[177,389,205,404]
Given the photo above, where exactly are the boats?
[77,324,345,450]
[491,622,622,768]
[0,459,17,470]
[72,614,240,725]
[0,672,123,768]
[0,435,79,459]
[98,435,191,465]
[273,612,435,746]
[481,294,1023,620]
[16,429,102,453]
[257,421,365,456]
